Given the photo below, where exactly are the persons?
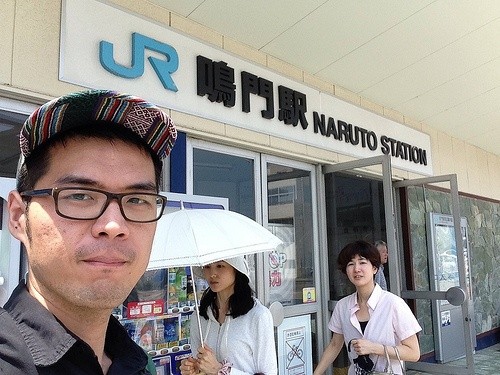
[312,242,423,375]
[179,255,277,375]
[0,89,177,375]
[373,240,388,291]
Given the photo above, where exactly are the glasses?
[375,240,383,246]
[18,185,168,225]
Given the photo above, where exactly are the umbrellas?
[146,200,283,375]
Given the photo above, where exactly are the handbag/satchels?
[362,344,407,375]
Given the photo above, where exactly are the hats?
[223,255,251,283]
[16,89,177,193]
